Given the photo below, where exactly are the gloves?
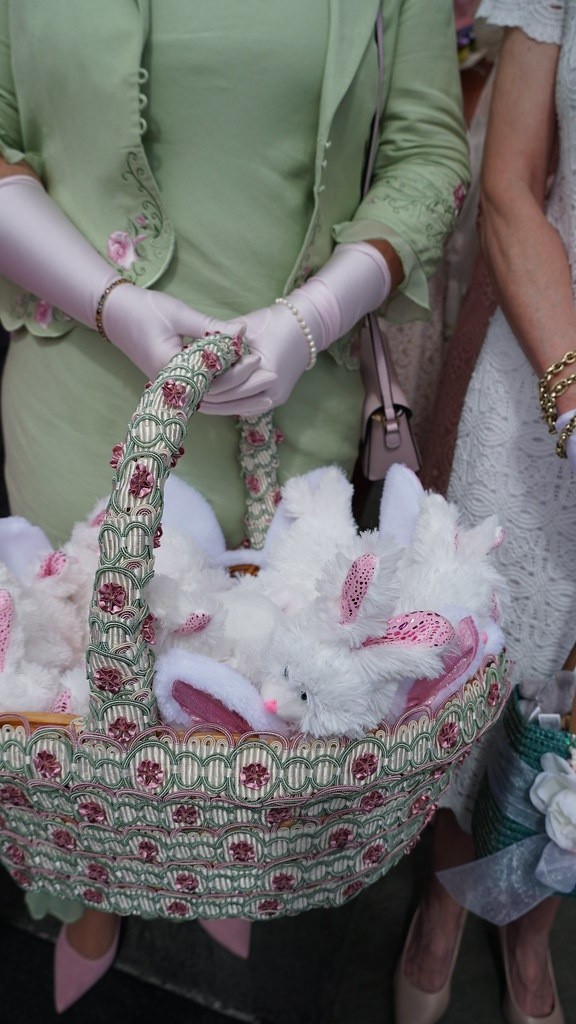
[0,173,280,416]
[181,241,390,417]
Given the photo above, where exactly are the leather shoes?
[198,913,255,963]
[42,909,118,1014]
[493,890,565,1023]
[390,880,471,1022]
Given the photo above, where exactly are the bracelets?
[95,278,135,342]
[274,297,317,372]
[535,349,576,462]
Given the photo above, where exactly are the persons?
[0,0,476,1015]
[395,0,575,1024]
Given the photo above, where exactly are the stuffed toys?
[0,460,514,775]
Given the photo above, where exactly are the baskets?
[0,333,513,926]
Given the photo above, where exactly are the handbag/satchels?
[357,312,422,483]
